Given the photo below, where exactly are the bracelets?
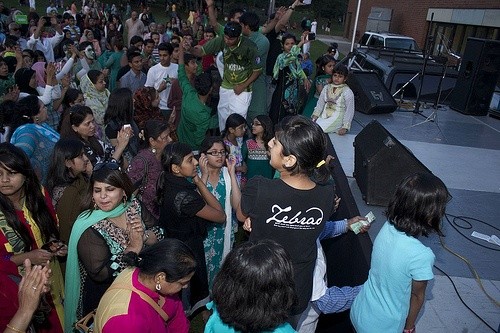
[403,327,415,333]
[7,325,26,333]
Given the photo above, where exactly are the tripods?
[397,12,453,143]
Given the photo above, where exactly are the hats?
[222,21,241,38]
[327,47,336,53]
[14,67,37,91]
[330,42,338,49]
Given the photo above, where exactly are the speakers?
[353,121,453,206]
[346,71,398,114]
[450,36,500,115]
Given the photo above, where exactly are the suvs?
[356,31,436,62]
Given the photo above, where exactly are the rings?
[32,286,37,289]
[127,134,128,137]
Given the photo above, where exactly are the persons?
[226,118,335,333]
[350,170,448,333]
[0,0,448,333]
[94,238,195,333]
[204,239,294,333]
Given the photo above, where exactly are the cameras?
[306,33,315,40]
[124,124,131,130]
[303,0,311,4]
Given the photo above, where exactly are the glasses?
[204,150,229,157]
[250,122,262,126]
[225,24,240,33]
[40,240,65,255]
